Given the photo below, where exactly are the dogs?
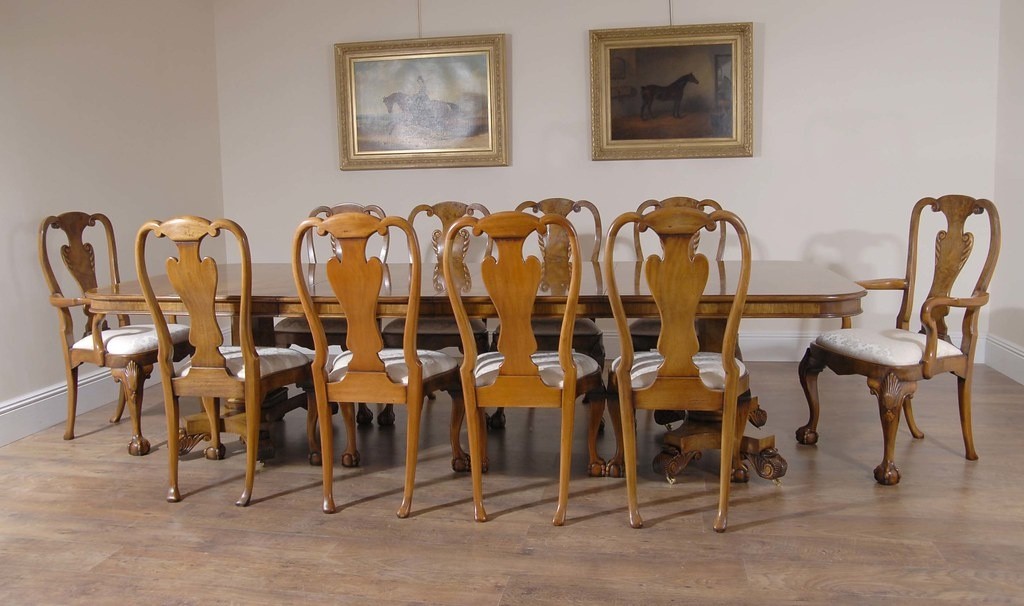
[639,72,700,119]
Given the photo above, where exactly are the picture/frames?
[333,33,509,171]
[589,22,754,162]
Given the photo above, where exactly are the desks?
[83,262,868,483]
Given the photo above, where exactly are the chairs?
[605,207,751,532]
[793,195,1002,484]
[134,216,322,506]
[490,198,605,434]
[442,216,607,527]
[37,212,192,457]
[290,216,467,518]
[377,199,495,429]
[275,201,374,425]
[630,198,727,342]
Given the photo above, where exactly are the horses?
[383,93,458,125]
